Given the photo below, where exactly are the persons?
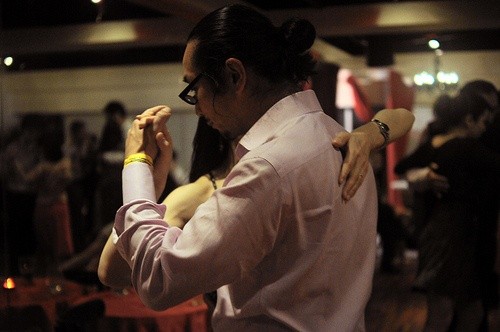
[111,3,378,332]
[0,100,127,278]
[394,80,500,332]
[97,105,415,332]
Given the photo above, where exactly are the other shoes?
[382,267,402,275]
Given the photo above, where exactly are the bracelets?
[370,118,389,147]
[123,152,154,167]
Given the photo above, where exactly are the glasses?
[178,68,205,106]
